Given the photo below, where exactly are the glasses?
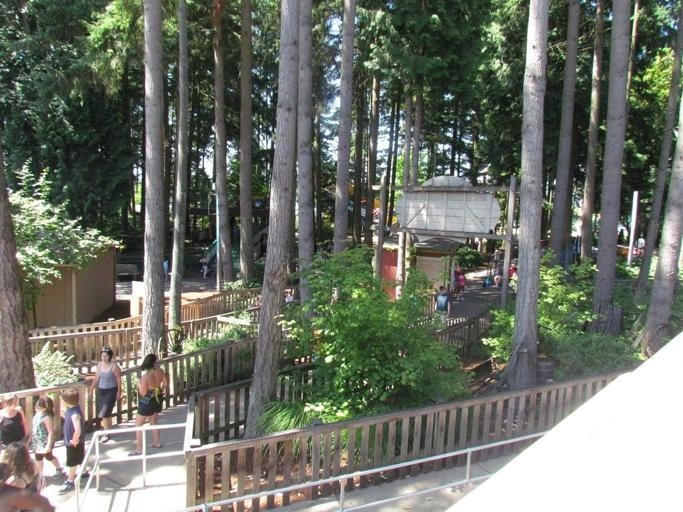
[101,347,112,352]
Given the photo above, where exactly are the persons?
[456,270,468,301]
[0,392,29,454]
[481,245,518,288]
[202,261,208,279]
[126,353,169,454]
[432,286,451,328]
[28,395,64,489]
[453,264,462,296]
[0,462,13,482]
[198,254,209,274]
[58,388,88,494]
[0,441,38,493]
[0,486,56,512]
[162,256,168,282]
[86,347,123,442]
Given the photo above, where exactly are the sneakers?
[80,470,89,478]
[58,480,76,494]
[54,468,63,479]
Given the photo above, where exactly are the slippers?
[102,434,163,456]
